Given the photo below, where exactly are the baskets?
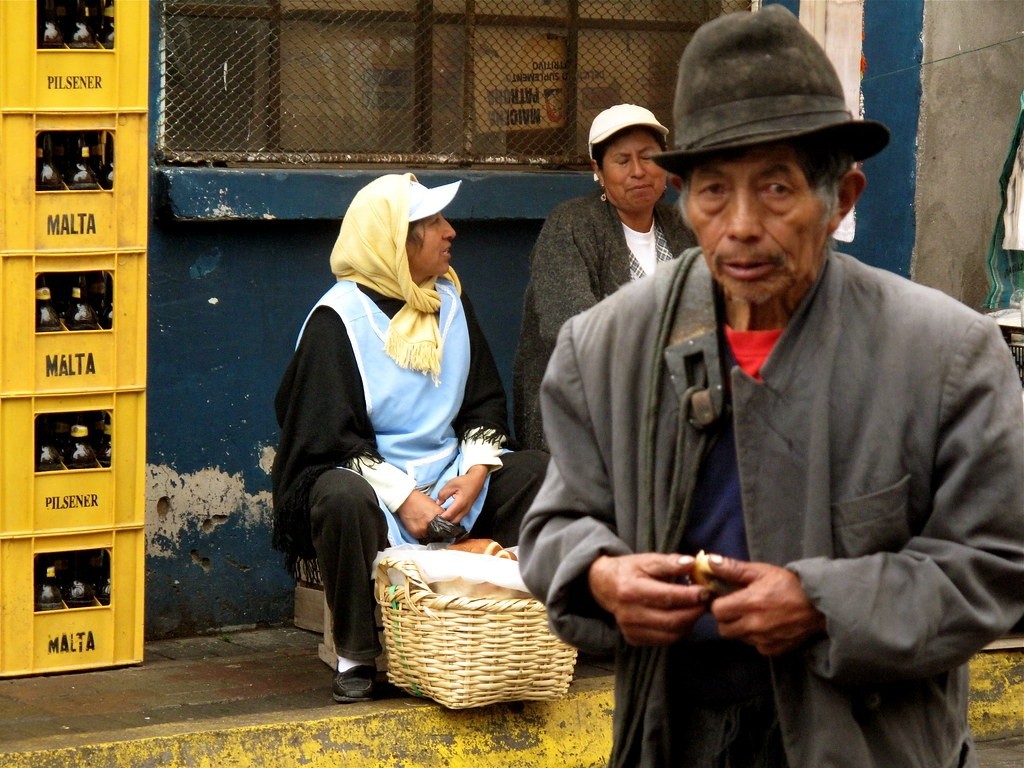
[375,558,578,710]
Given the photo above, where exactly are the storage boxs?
[0,389,146,536]
[0,525,144,677]
[0,0,151,114]
[0,113,148,254]
[0,252,147,396]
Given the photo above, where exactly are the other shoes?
[330,659,376,702]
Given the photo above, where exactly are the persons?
[269,170,552,701]
[509,102,700,457]
[514,1,1024,768]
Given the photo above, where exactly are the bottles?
[35,411,111,472]
[36,130,113,190]
[34,549,110,612]
[35,272,113,332]
[38,0,114,50]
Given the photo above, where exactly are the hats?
[407,178,462,224]
[587,104,670,160]
[647,3,891,177]
